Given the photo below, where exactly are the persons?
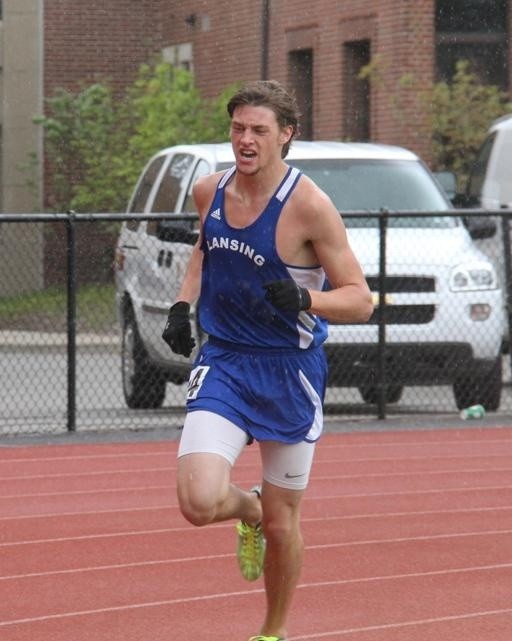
[162,80,374,641]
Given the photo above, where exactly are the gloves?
[162,301,195,358]
[261,278,313,315]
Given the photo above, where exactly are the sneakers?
[236,486,266,583]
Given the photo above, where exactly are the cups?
[461,404,485,420]
